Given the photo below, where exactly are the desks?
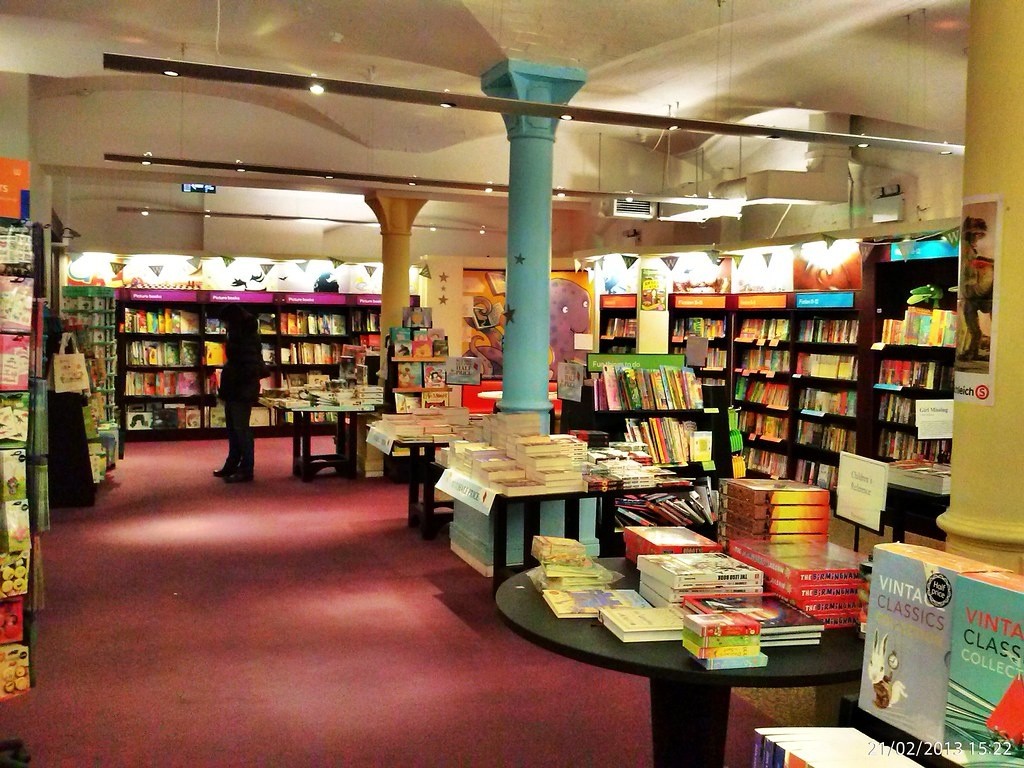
[495,557,864,768]
[430,461,694,573]
[257,396,377,483]
[845,479,949,553]
[365,422,466,541]
[478,391,559,434]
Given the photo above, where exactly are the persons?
[213,305,271,483]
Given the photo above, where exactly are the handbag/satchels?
[256,353,271,378]
[45,332,90,395]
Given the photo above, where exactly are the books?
[435,413,586,515]
[673,318,726,368]
[569,417,698,487]
[395,392,448,413]
[594,363,725,410]
[528,479,1024,768]
[0,156,51,703]
[876,306,959,495]
[793,320,860,492]
[398,357,480,388]
[389,308,449,356]
[366,407,468,455]
[125,308,384,431]
[606,318,637,352]
[62,297,118,483]
[729,319,791,478]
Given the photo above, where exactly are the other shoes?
[213,463,239,477]
[224,466,254,483]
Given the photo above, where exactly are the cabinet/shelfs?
[861,240,959,542]
[276,291,350,434]
[62,284,116,422]
[48,389,94,508]
[557,385,734,490]
[599,293,638,354]
[115,288,202,442]
[348,293,420,384]
[199,289,279,438]
[727,290,793,479]
[788,288,861,510]
[667,292,730,404]
[390,354,453,393]
[382,333,449,413]
